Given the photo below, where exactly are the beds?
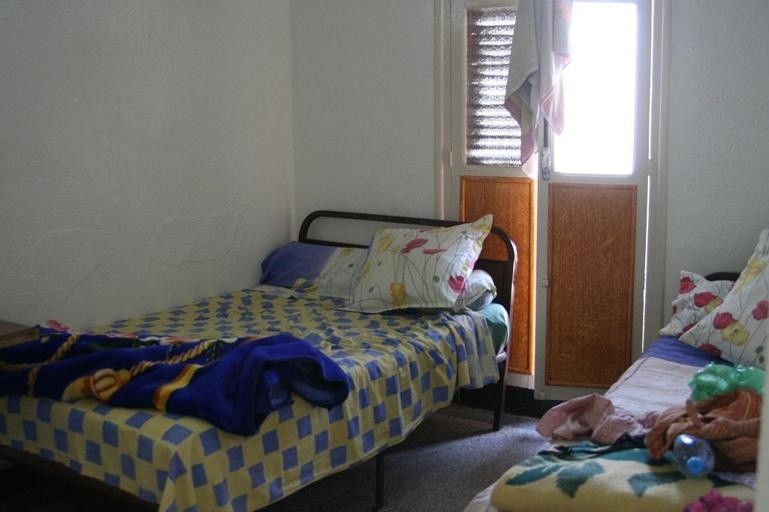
[1,209,518,512]
[465,272,769,512]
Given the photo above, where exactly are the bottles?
[673,433,715,478]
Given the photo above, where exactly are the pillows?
[657,229,769,372]
[256,214,498,313]
